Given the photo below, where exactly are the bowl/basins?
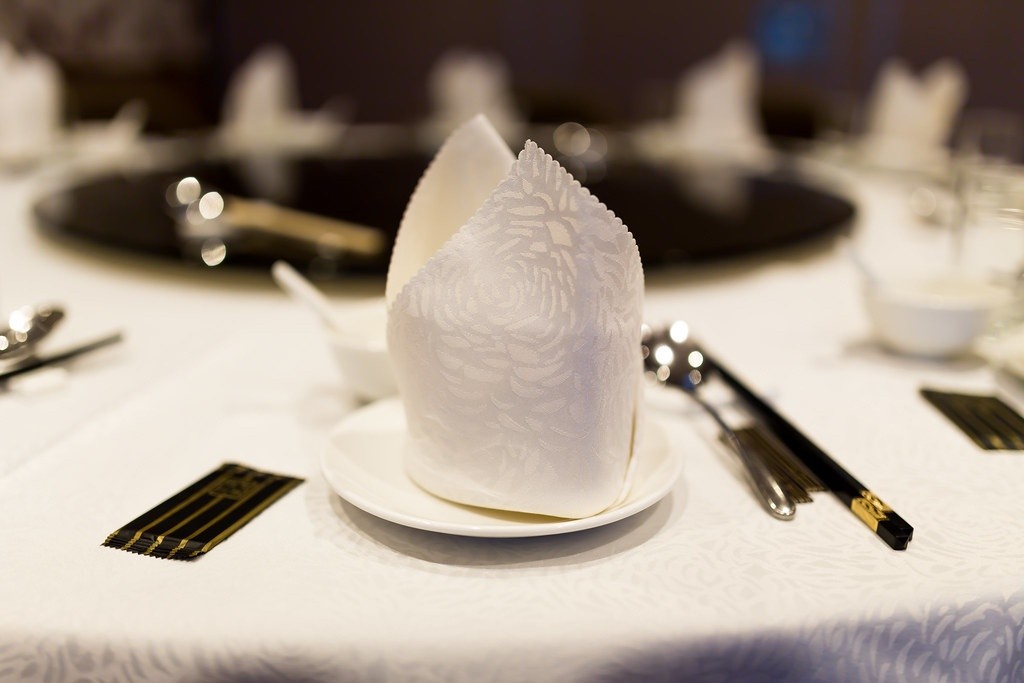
[867,283,1009,361]
[324,330,398,405]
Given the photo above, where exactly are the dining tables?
[0,123,1024,683]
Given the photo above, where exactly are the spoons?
[640,330,796,521]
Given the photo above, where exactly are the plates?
[319,392,689,538]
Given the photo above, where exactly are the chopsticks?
[699,344,915,551]
